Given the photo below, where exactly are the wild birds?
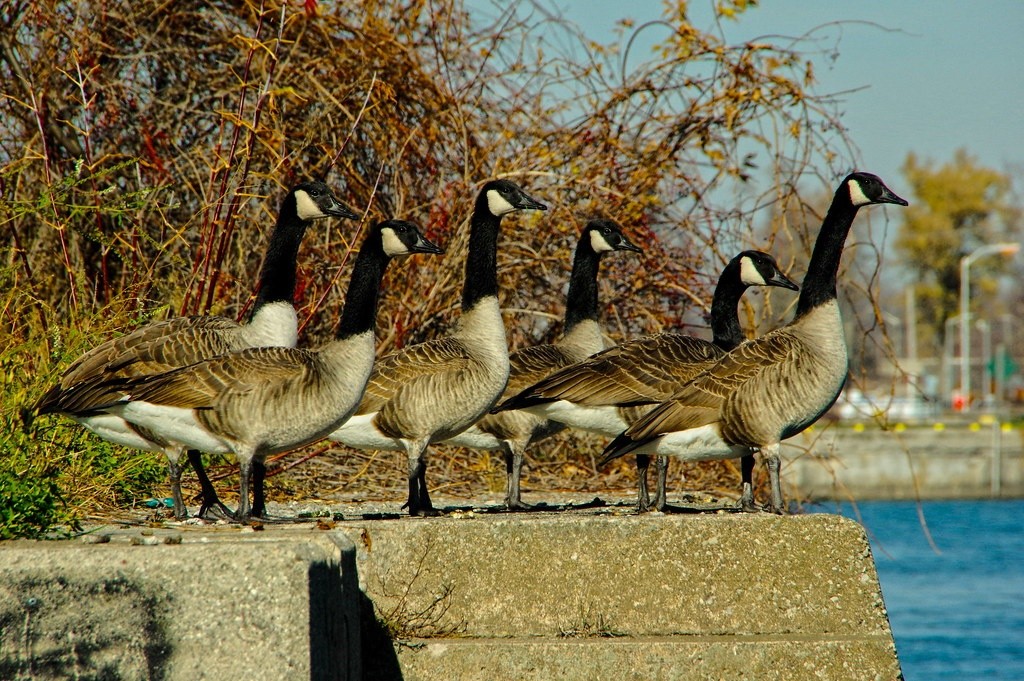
[21,169,914,522]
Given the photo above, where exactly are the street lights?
[960,242,1020,412]
